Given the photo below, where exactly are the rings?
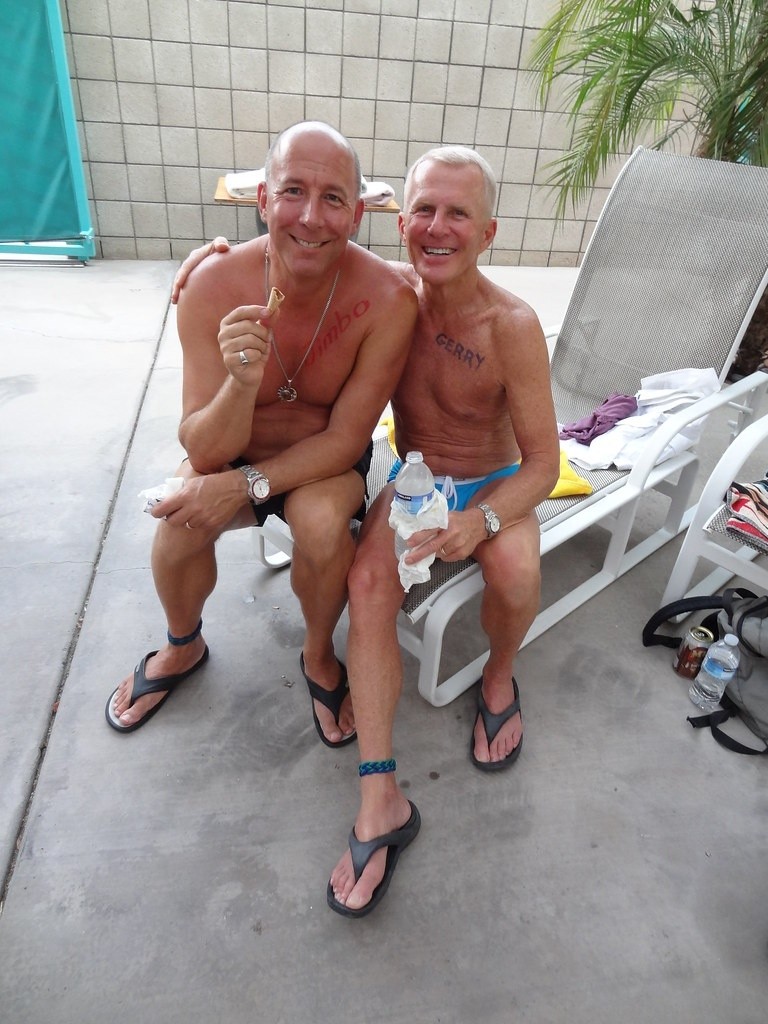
[238,351,249,365]
[187,522,193,530]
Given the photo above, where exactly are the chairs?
[252,144,768,708]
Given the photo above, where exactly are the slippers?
[105,641,208,733]
[469,674,523,772]
[299,650,359,749]
[326,796,421,918]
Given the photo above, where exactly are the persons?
[167,144,561,920]
[105,117,422,748]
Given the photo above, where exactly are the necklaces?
[264,238,339,403]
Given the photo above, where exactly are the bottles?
[689,634,741,713]
[395,451,435,561]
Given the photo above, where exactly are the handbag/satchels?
[641,586,768,755]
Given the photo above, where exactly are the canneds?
[672,626,714,679]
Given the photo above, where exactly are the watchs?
[473,501,501,540]
[238,465,272,505]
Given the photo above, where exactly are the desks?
[214,176,400,238]
